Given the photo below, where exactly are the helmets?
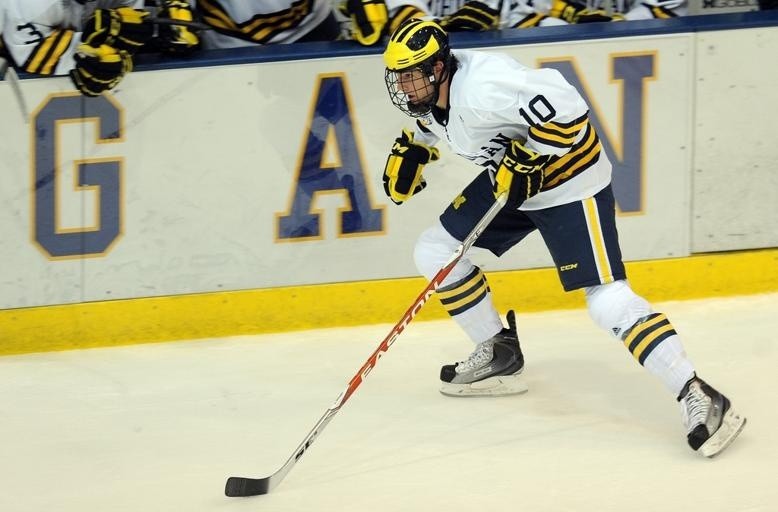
[384,19,451,72]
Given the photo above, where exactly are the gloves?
[492,141,549,209]
[69,1,198,98]
[382,129,439,203]
[343,2,627,45]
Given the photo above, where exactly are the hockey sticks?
[224,192,509,498]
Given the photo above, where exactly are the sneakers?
[676,369,732,451]
[441,310,524,383]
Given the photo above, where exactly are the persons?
[382,17,732,452]
